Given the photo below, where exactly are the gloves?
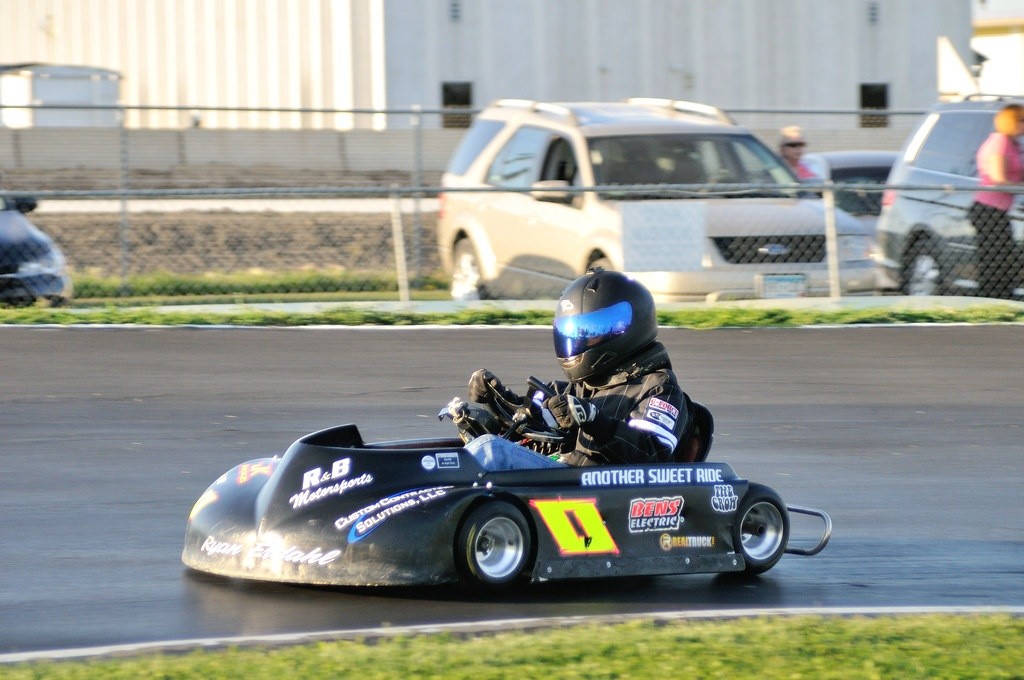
[542,394,598,429]
[468,368,506,404]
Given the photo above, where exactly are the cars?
[0,190,71,311]
[800,151,904,231]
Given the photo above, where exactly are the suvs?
[435,97,887,304]
[876,90,1023,299]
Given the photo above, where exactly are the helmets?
[552,267,658,385]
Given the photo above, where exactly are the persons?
[769,124,816,185]
[968,105,1024,297]
[462,266,689,471]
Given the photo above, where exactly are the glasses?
[780,142,804,148]
